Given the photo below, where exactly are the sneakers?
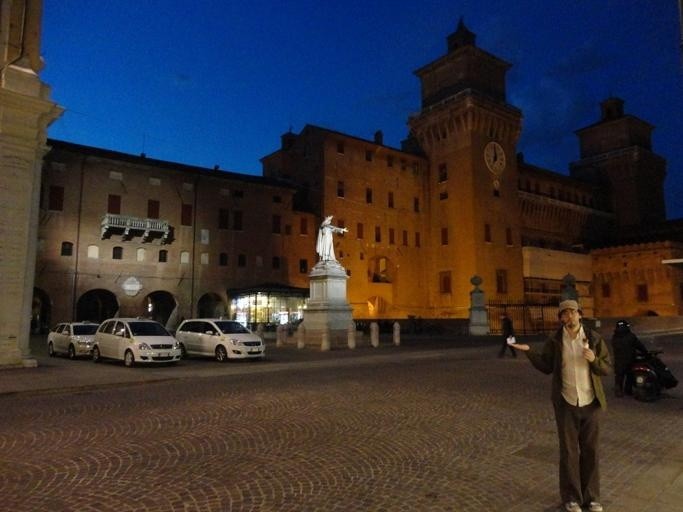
[565,502,582,512]
[588,502,602,512]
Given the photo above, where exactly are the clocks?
[484,142,506,175]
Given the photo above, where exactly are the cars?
[43,318,101,361]
[88,315,182,369]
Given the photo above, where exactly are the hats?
[559,300,582,315]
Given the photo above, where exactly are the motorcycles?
[631,349,665,401]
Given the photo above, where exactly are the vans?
[173,317,269,364]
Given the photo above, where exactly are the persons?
[316,215,350,262]
[496,314,518,359]
[611,320,648,398]
[508,299,609,512]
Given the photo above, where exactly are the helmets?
[616,321,630,329]
